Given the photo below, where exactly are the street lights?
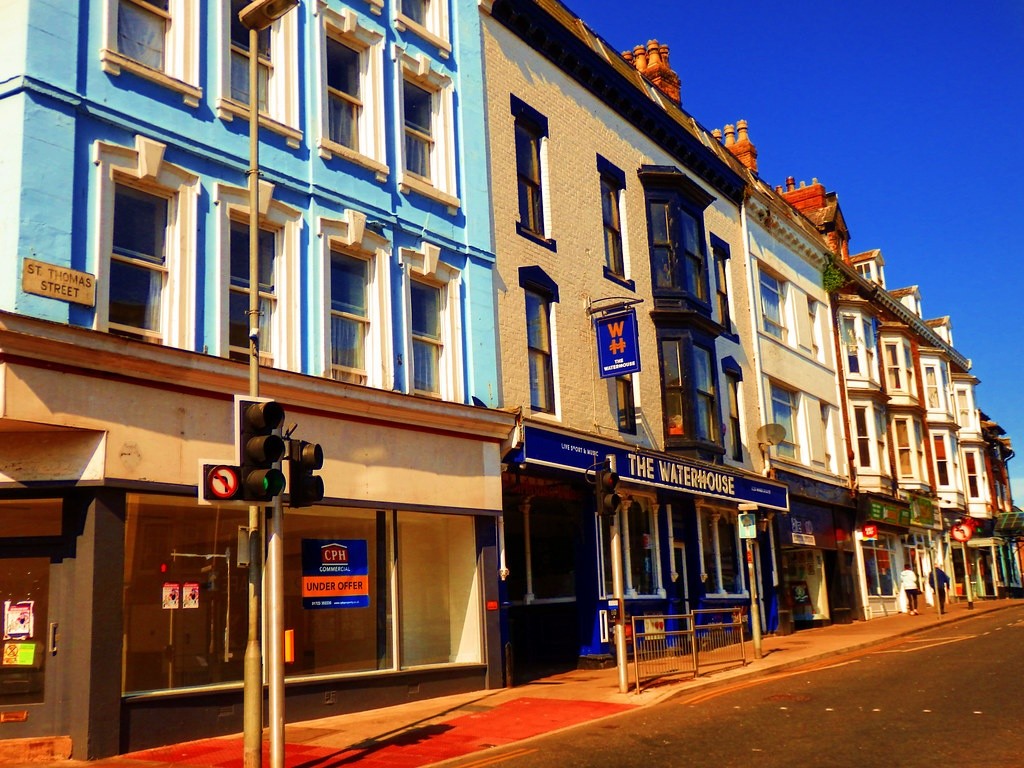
[236,0,303,767]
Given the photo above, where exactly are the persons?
[900,564,919,616]
[929,563,950,614]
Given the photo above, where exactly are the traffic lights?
[233,394,287,506]
[595,469,620,516]
[288,439,324,507]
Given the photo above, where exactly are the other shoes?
[910,609,919,615]
